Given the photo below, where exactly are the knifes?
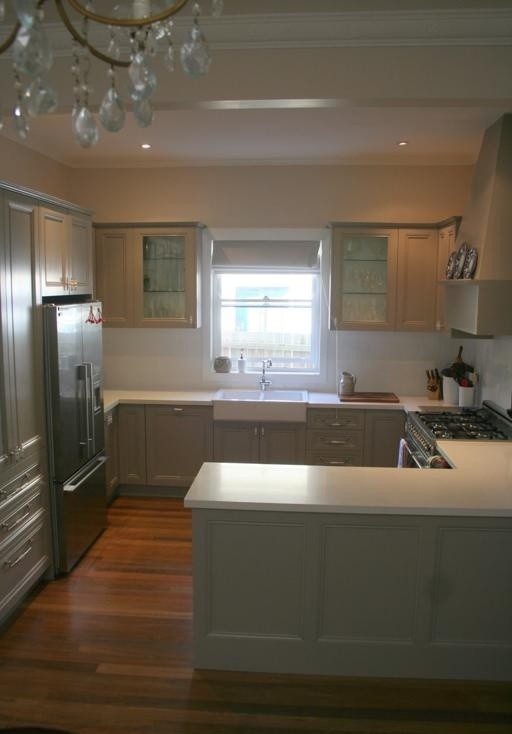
[431,369,434,378]
[435,368,439,379]
[426,369,429,380]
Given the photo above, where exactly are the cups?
[459,385,475,406]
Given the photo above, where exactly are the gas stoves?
[406,400,511,461]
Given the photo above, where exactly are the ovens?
[401,438,433,468]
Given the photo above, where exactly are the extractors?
[437,112,510,337]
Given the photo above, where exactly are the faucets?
[259,359,273,387]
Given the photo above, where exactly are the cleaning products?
[237,346,246,372]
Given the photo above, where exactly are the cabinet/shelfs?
[214,423,306,464]
[362,407,405,467]
[119,404,215,488]
[327,221,437,334]
[39,200,93,299]
[308,409,367,467]
[436,218,462,330]
[93,221,204,329]
[104,404,120,505]
[0,182,56,615]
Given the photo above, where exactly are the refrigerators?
[44,299,108,577]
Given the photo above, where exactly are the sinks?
[212,387,308,425]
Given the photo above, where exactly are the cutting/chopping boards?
[338,390,399,403]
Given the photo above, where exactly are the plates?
[454,242,465,278]
[446,252,456,278]
[464,249,478,279]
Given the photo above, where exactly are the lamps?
[1,1,224,148]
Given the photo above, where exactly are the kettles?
[338,369,358,395]
